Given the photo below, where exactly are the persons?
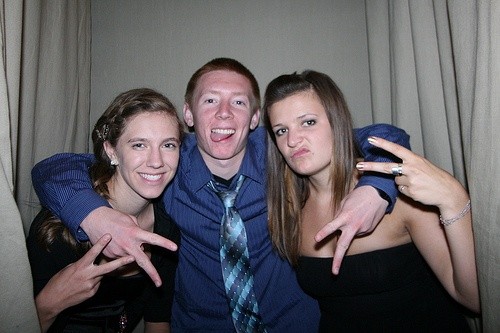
[30,57,413,333]
[27,88,180,333]
[258,70,482,333]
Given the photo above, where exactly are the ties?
[205,175,268,333]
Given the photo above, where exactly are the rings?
[392,164,402,175]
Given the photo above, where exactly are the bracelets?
[440,201,469,227]
